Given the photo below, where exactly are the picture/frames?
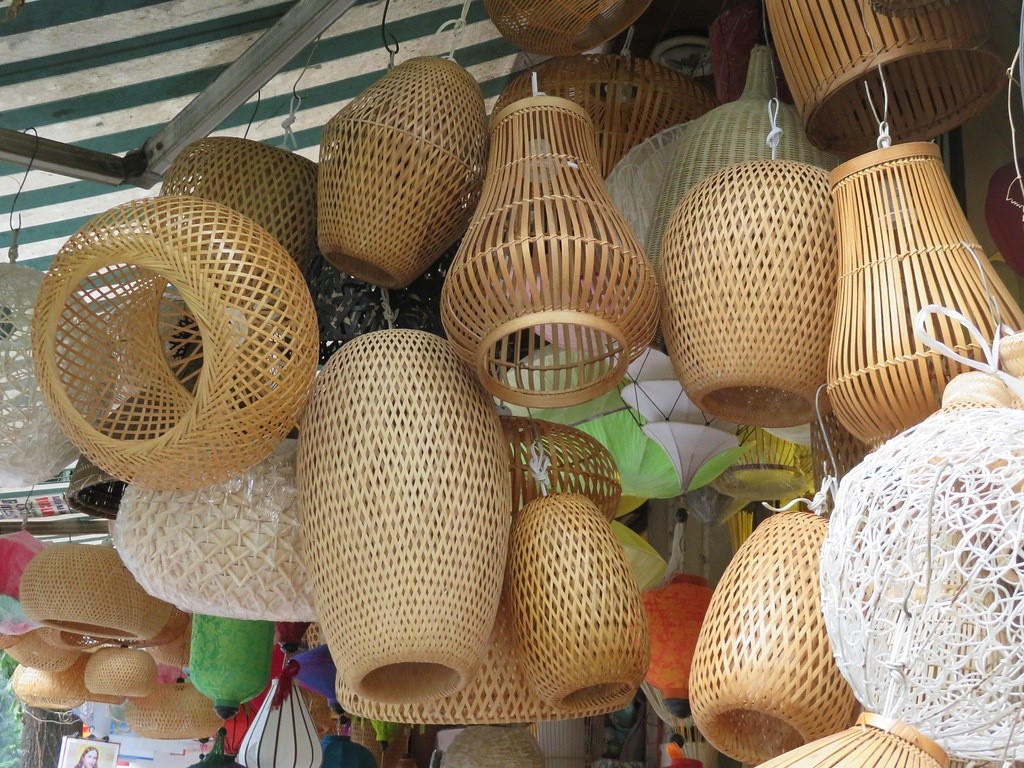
[58,737,119,768]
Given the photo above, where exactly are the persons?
[73,746,99,768]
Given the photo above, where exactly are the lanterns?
[0,0,1024,768]
